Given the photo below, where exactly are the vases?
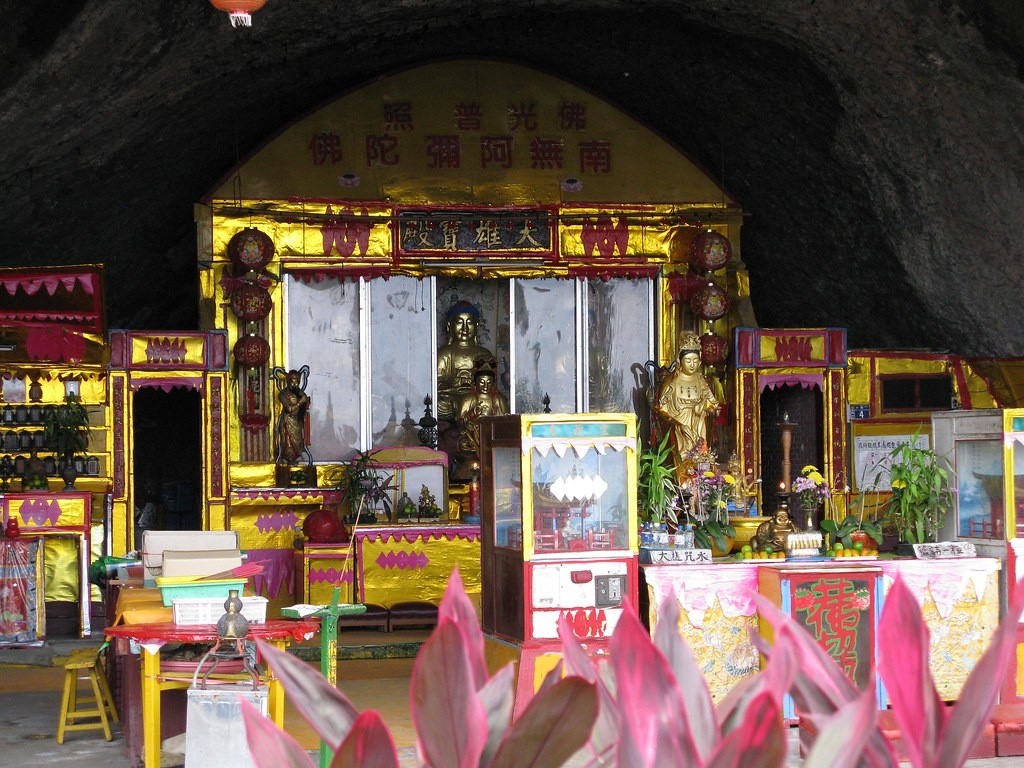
[893,542,914,556]
[694,535,737,557]
[804,509,818,530]
[837,530,867,547]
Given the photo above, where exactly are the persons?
[658,330,723,488]
[399,492,414,514]
[437,300,510,483]
[275,370,308,465]
[750,510,802,553]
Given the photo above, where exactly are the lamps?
[774,411,799,521]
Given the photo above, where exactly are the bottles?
[685,526,694,548]
[639,523,669,547]
[674,526,685,548]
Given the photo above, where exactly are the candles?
[470,482,480,517]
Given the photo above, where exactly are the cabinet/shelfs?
[932,407,1024,757]
[0,401,114,483]
[479,413,639,697]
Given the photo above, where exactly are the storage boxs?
[172,597,269,625]
[141,531,249,607]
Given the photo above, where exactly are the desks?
[352,525,481,623]
[639,558,1001,729]
[103,578,322,768]
[293,542,355,605]
[4,490,92,639]
[0,535,47,649]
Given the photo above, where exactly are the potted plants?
[43,401,96,491]
[326,448,400,524]
[636,427,684,548]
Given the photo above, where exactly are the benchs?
[57,648,119,744]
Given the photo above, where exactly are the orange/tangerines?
[825,542,879,558]
[735,545,785,560]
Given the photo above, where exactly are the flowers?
[819,463,883,545]
[686,468,735,552]
[868,421,957,545]
[792,464,831,508]
